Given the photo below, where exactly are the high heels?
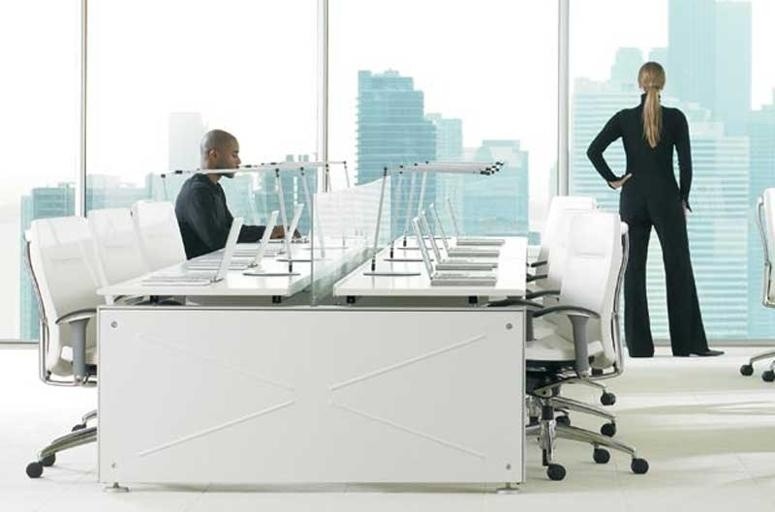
[671,345,725,357]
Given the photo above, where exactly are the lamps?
[161,160,506,275]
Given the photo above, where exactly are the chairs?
[480,195,648,481]
[739,189,775,382]
[22,201,189,477]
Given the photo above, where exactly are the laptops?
[428,203,499,257]
[445,196,505,246]
[142,216,243,285]
[411,216,495,286]
[420,212,492,271]
[185,210,278,273]
[226,203,306,255]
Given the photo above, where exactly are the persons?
[586,61,726,357]
[174,127,302,260]
